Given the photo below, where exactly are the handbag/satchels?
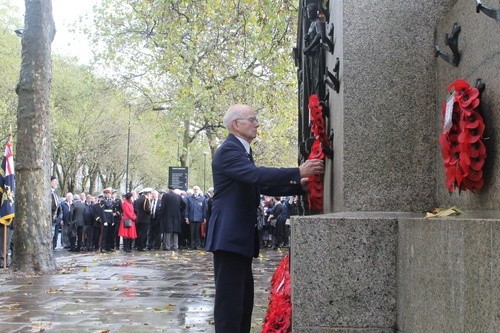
[124,220,132,228]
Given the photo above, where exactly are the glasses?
[236,117,258,123]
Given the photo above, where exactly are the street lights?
[202,151,209,194]
[123,100,137,195]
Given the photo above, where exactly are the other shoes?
[68,248,80,252]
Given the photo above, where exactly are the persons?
[51,176,61,236]
[257,194,298,249]
[204,104,325,333]
[54,186,214,253]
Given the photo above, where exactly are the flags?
[0,134,15,226]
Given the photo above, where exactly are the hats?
[103,190,110,193]
[144,191,151,194]
[168,185,175,189]
[126,192,132,197]
